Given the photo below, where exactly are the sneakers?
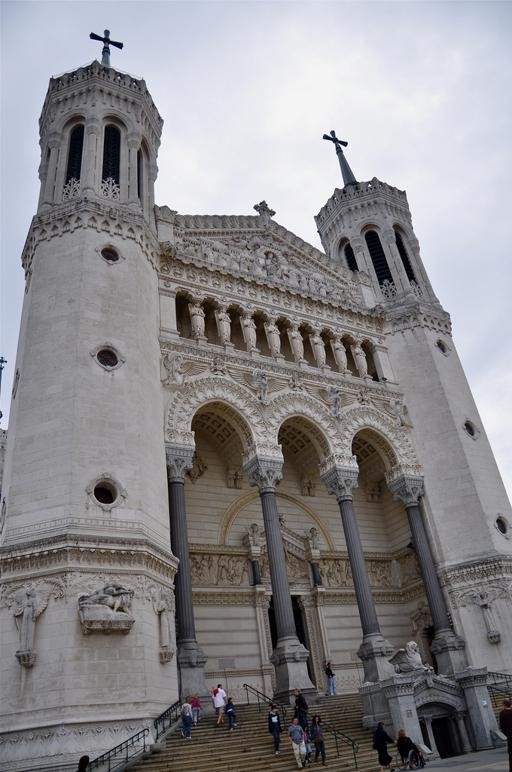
[275,751,281,756]
[180,730,185,739]
[233,723,238,727]
[229,728,234,732]
[184,737,192,740]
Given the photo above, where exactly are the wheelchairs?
[396,743,426,770]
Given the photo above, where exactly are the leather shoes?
[314,757,319,762]
[322,761,329,766]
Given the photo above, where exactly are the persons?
[180,684,238,739]
[397,729,425,763]
[325,662,336,696]
[269,688,326,770]
[373,722,398,772]
[499,698,510,771]
